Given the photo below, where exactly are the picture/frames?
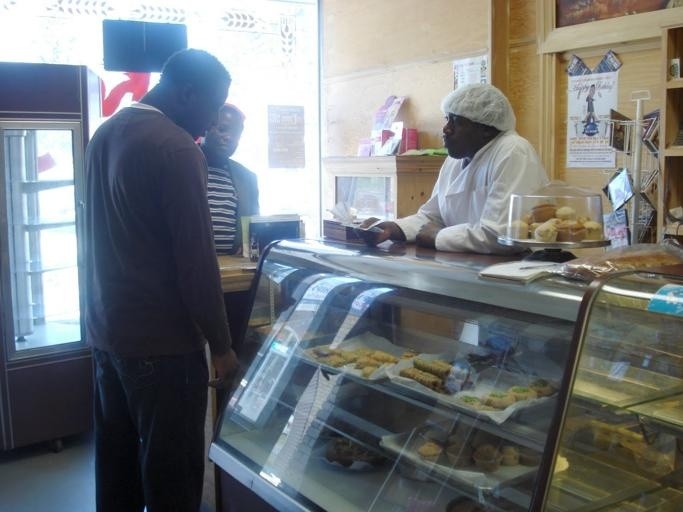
[536,1,683,54]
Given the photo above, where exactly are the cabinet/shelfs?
[205,239,683,512]
[1,63,103,457]
[655,6,683,247]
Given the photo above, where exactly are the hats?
[443,83,516,132]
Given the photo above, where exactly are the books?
[478,261,562,286]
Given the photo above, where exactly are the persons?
[198,101,259,255]
[358,83,548,256]
[80,49,238,512]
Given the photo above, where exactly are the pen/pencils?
[519,262,556,269]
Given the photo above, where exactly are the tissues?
[322,202,367,244]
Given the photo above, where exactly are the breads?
[301,345,555,473]
[509,203,602,241]
